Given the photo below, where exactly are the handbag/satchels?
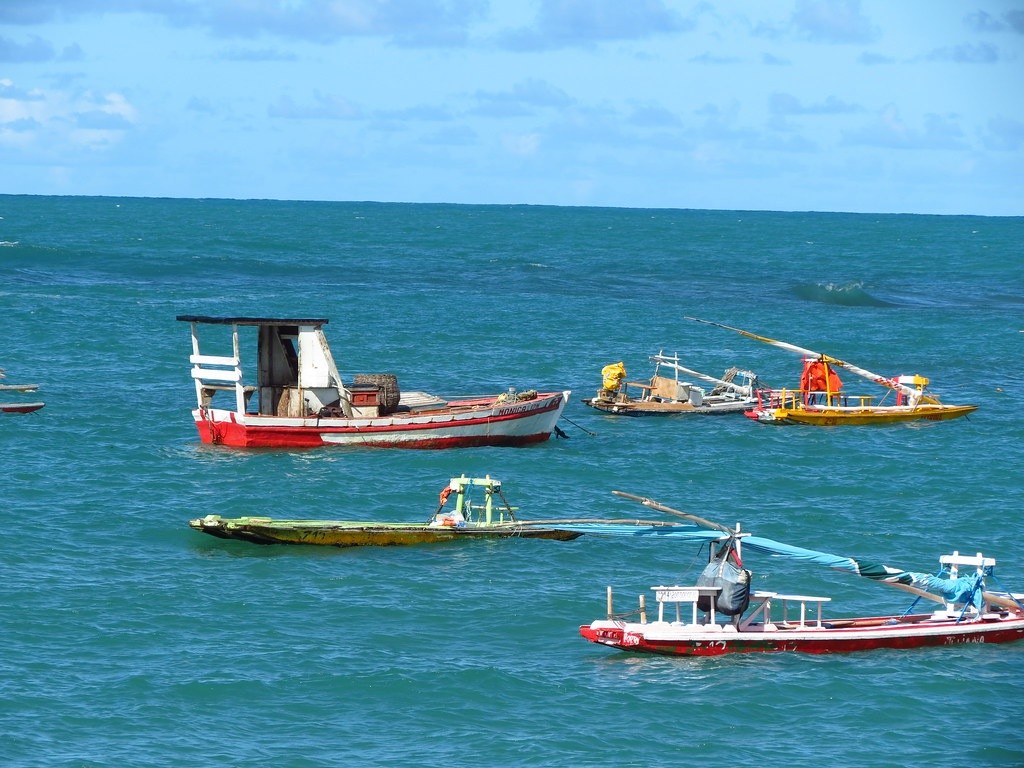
[695,535,751,616]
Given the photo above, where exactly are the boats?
[189,473,585,544]
[176,314,570,447]
[585,350,796,417]
[749,354,981,425]
[577,521,1024,658]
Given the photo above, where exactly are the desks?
[623,382,657,400]
[201,383,258,412]
[651,586,723,623]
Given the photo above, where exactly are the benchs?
[806,392,876,406]
[744,591,831,630]
